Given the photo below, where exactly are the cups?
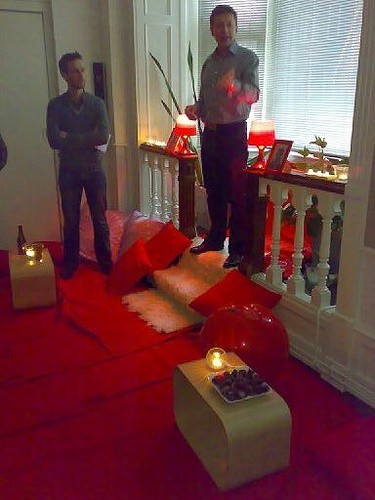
[33,243,43,263]
[24,244,36,267]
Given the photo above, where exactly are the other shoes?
[189,238,224,253]
[59,258,80,278]
[222,254,245,268]
[97,256,113,274]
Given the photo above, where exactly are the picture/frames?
[265,138,294,174]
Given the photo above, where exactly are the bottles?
[17,224,26,255]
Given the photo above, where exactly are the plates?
[208,366,272,403]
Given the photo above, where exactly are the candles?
[24,245,36,258]
[205,347,228,370]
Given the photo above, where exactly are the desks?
[8,246,57,311]
[171,352,295,494]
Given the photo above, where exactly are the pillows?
[109,237,153,296]
[147,220,191,274]
[77,200,130,264]
[189,270,282,322]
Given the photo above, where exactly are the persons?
[46,51,113,279]
[270,146,285,170]
[185,5,260,268]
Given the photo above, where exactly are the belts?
[204,124,229,131]
